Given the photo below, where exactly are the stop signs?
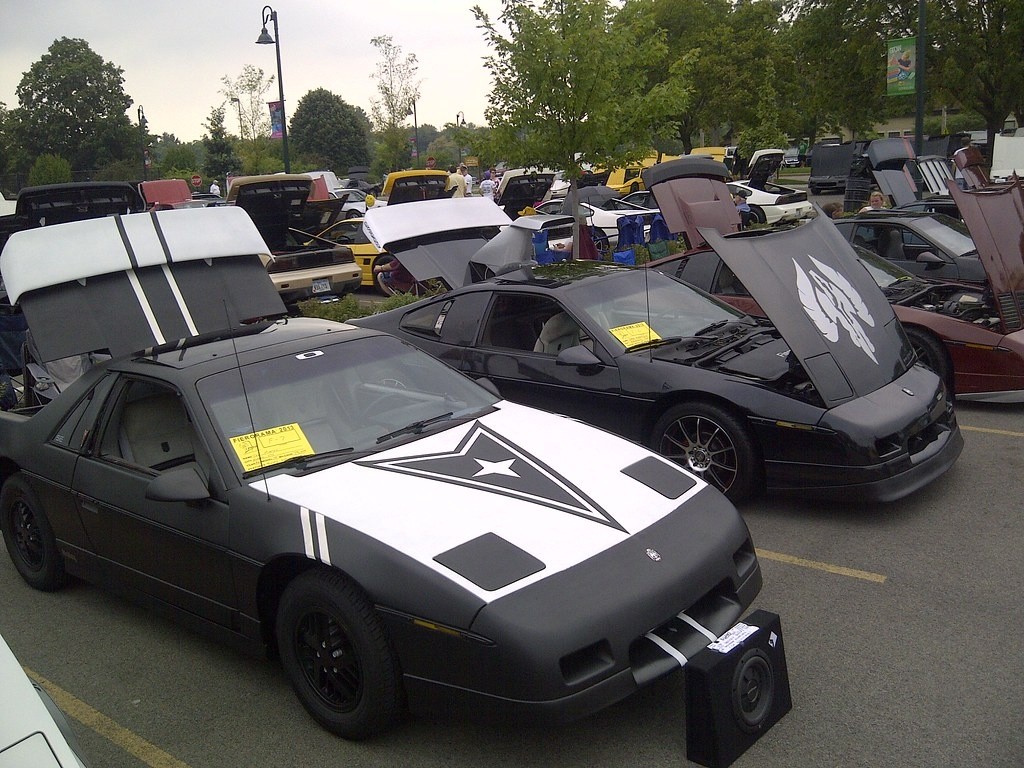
[426,156,437,170]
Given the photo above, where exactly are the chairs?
[582,289,609,335]
[716,267,736,295]
[120,391,213,481]
[878,229,907,261]
[534,312,581,355]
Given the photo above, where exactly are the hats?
[732,190,747,200]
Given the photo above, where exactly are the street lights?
[255,4,293,176]
[406,96,422,171]
[230,96,244,150]
[456,110,467,165]
[135,105,150,181]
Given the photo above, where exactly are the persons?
[730,190,751,231]
[209,179,221,197]
[859,192,887,212]
[425,157,472,198]
[479,168,500,198]
[733,143,748,180]
[952,137,973,190]
[822,202,845,220]
[897,50,914,80]
[374,258,420,297]
[797,140,807,167]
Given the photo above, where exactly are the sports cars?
[614,155,1024,403]
[0,204,801,768]
[349,201,967,519]
[0,129,1023,310]
